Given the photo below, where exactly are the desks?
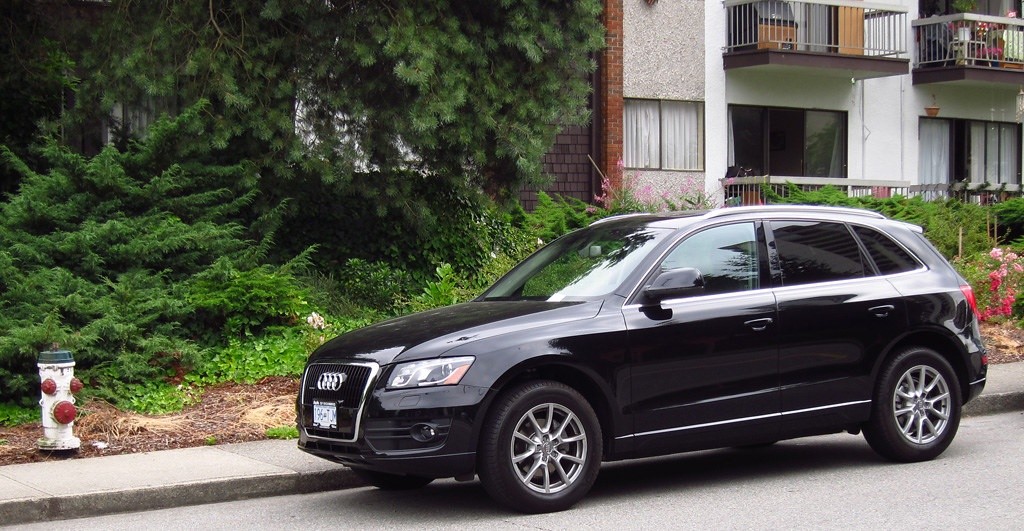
[943,41,992,68]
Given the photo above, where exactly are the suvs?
[297,204,991,511]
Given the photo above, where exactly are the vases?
[924,107,940,117]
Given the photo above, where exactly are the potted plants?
[953,0,974,41]
